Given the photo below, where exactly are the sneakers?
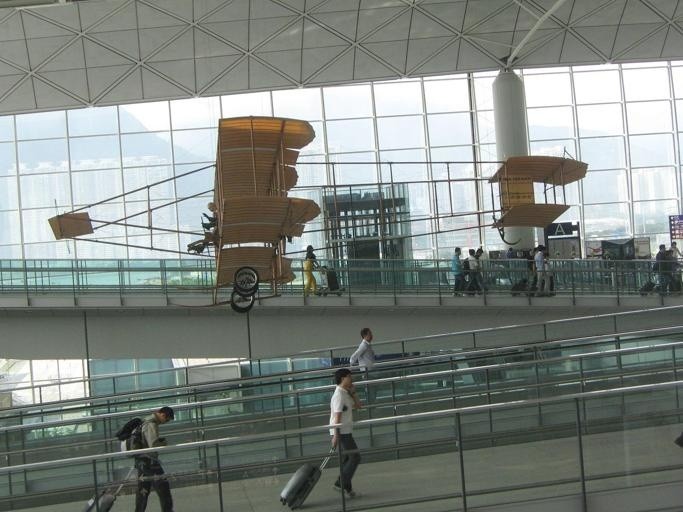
[344,490,356,499]
[333,482,342,491]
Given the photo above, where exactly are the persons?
[132,406,174,512]
[304,246,320,297]
[350,327,376,404]
[452,244,556,298]
[329,369,361,498]
[654,241,683,295]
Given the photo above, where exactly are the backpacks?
[119,417,154,457]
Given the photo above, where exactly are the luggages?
[82,467,136,511]
[279,449,335,510]
[322,269,339,290]
[512,279,529,296]
[641,281,655,294]
